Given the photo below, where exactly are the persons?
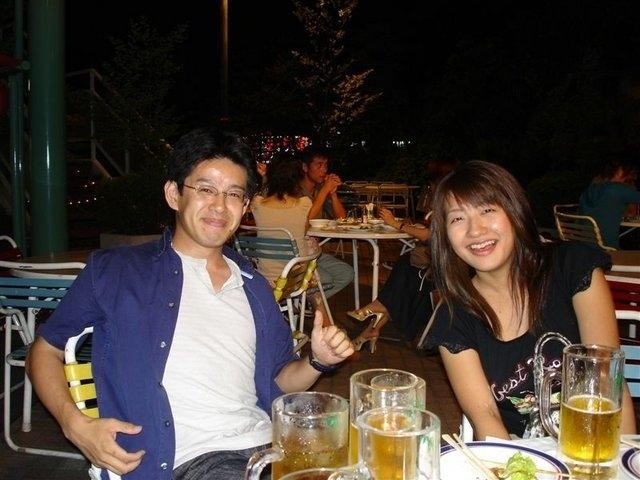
[296,144,355,319]
[428,159,637,442]
[346,163,459,355]
[248,151,313,322]
[25,130,355,480]
[574,157,640,251]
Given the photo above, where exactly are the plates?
[309,216,404,229]
[621,446,640,479]
[436,440,570,480]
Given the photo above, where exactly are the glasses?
[179,183,250,205]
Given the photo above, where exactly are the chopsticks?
[442,429,506,480]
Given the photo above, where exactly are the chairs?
[539,201,640,402]
[0,233,123,480]
[238,177,448,355]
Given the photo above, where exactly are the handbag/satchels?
[521,330,576,438]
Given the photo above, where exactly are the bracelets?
[399,223,405,230]
[308,349,337,373]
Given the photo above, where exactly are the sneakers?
[292,299,313,317]
[280,310,299,322]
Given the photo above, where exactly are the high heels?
[351,335,378,354]
[346,304,385,329]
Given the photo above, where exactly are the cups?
[538,341,624,479]
[243,369,441,480]
